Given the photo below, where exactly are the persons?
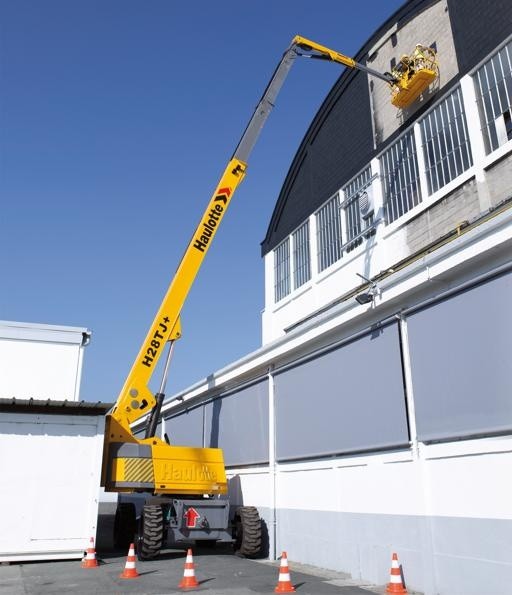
[401,43,430,73]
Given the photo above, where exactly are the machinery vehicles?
[102,35,438,562]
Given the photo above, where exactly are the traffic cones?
[119,543,141,579]
[274,552,296,593]
[388,554,406,595]
[179,548,198,588]
[81,538,98,569]
